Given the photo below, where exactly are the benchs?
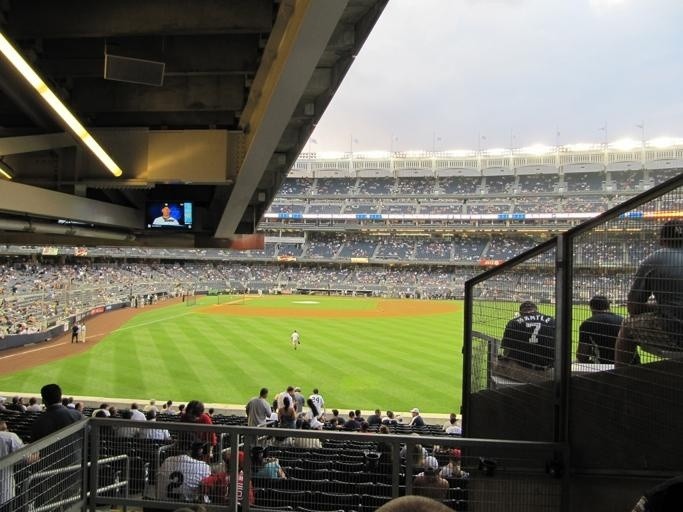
[491,362,617,388]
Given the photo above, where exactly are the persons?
[613,219,682,368]
[575,294,641,366]
[0,383,501,511]
[288,329,300,350]
[263,265,682,308]
[151,203,181,227]
[0,244,263,344]
[488,300,555,374]
[264,171,683,262]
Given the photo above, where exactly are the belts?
[514,360,551,371]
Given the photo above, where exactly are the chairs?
[0,401,470,512]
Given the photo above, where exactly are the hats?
[409,408,419,413]
[162,202,170,209]
[423,456,438,472]
[660,219,682,238]
[520,301,537,312]
